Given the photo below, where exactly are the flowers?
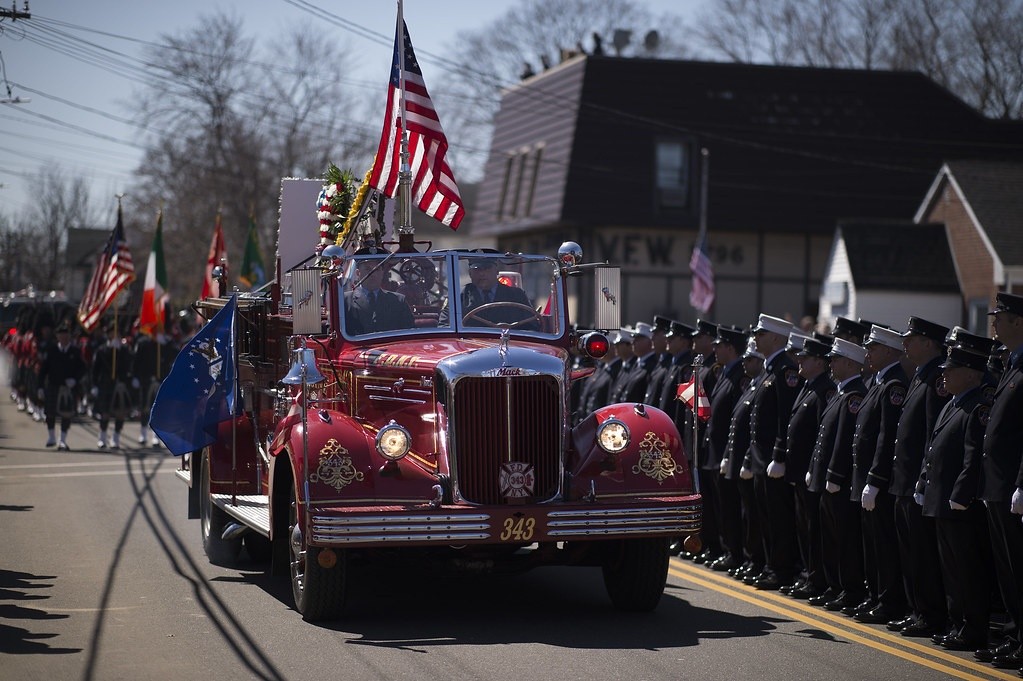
[318,163,356,247]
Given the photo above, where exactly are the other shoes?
[59,443,69,451]
[46,442,56,446]
[97,436,160,450]
[16,402,46,421]
[77,402,101,420]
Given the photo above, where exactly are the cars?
[168,0,705,630]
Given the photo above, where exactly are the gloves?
[914,493,924,506]
[805,471,813,486]
[949,500,968,510]
[861,483,879,511]
[739,465,753,479]
[825,480,840,493]
[720,457,729,474]
[1010,487,1023,514]
[766,460,785,478]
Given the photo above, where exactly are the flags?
[196,216,227,325]
[149,294,242,457]
[75,208,137,334]
[238,212,268,292]
[140,215,173,346]
[368,12,465,233]
[689,233,715,312]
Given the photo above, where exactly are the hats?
[603,316,746,344]
[898,317,949,343]
[937,325,994,373]
[985,291,1023,315]
[467,248,499,269]
[741,313,904,365]
[354,248,388,262]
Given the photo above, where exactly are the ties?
[482,290,490,304]
[942,399,956,421]
[366,293,375,305]
[1003,357,1013,380]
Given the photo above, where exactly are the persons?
[569,293,1023,676]
[437,248,540,331]
[344,246,415,337]
[2,308,197,451]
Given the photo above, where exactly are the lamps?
[602,287,616,305]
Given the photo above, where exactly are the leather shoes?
[679,548,1023,678]
